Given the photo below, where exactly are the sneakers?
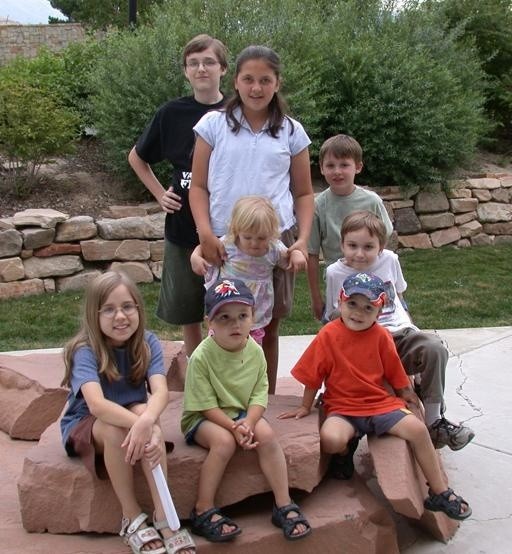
[429,416,474,450]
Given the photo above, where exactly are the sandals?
[272,500,312,540]
[119,510,197,554]
[190,507,241,541]
[424,487,472,519]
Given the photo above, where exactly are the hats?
[204,278,254,320]
[340,272,385,307]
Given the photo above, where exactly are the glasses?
[98,303,139,318]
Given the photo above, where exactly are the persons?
[190,196,307,347]
[188,46,314,395]
[181,279,311,542]
[275,272,472,520]
[128,34,233,357]
[61,272,196,554]
[307,135,409,320]
[323,212,475,450]
